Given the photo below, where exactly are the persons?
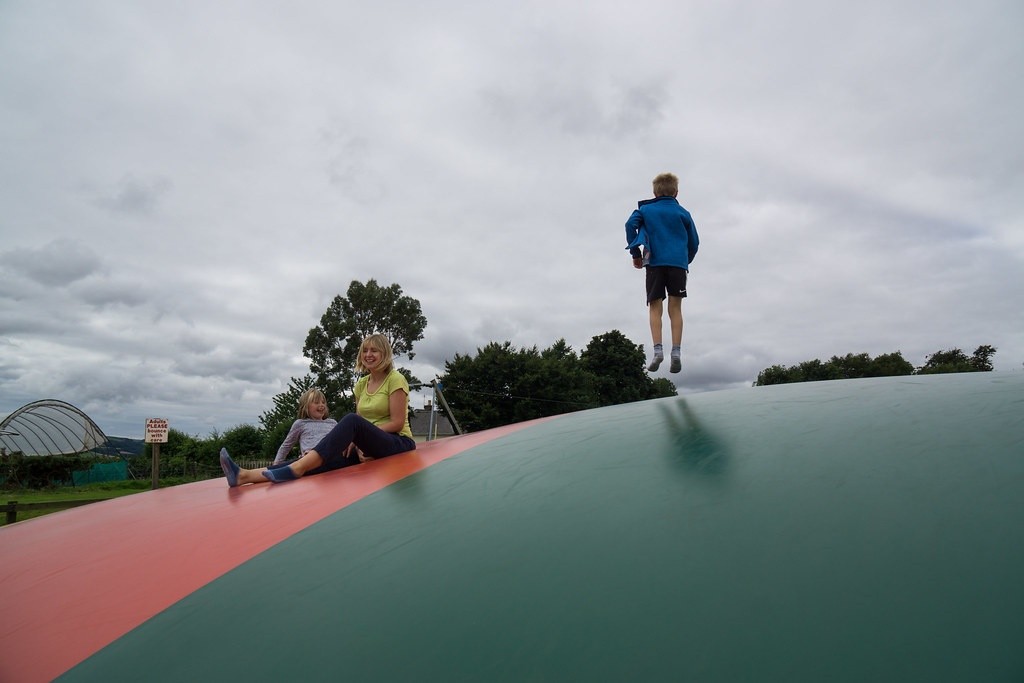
[624,173,699,373]
[269,388,361,475]
[219,334,416,487]
